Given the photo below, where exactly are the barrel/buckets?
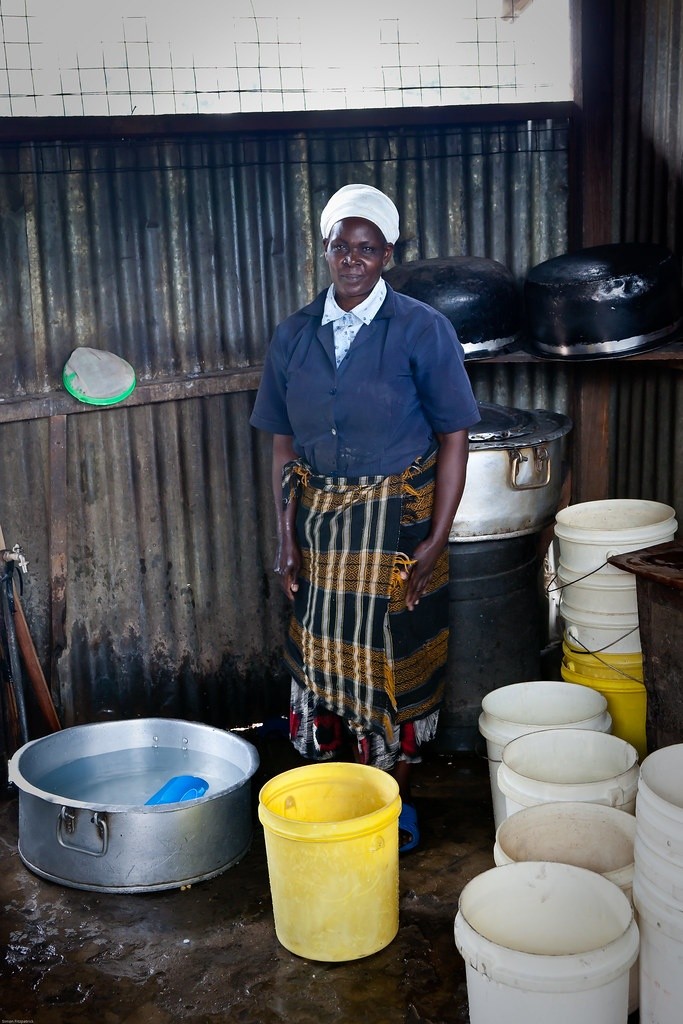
[442,499,683,1023]
[258,762,402,962]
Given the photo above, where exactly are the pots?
[382,255,521,362]
[523,242,683,361]
[8,718,258,893]
[447,401,574,544]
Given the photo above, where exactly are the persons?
[249,184,469,854]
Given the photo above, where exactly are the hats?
[320,184,399,245]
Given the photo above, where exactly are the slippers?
[399,803,419,851]
